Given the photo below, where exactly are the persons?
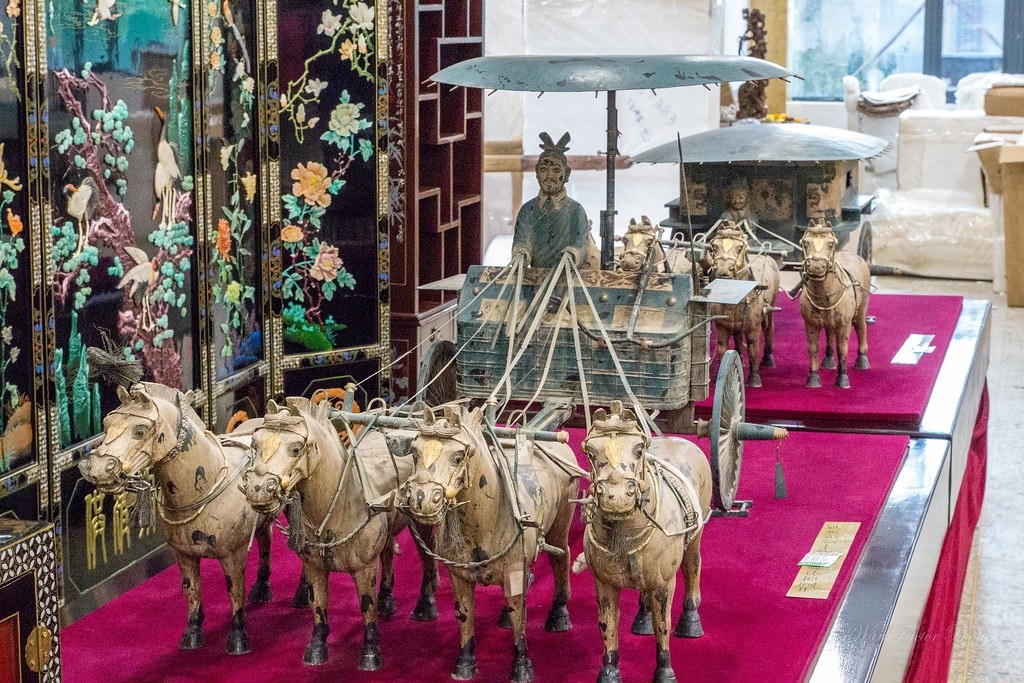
[511,132,588,270]
[718,175,757,234]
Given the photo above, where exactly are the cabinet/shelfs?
[224,0,485,405]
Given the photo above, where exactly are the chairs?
[842,71,1024,191]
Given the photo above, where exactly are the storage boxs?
[964,128,1024,308]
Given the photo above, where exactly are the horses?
[239,393,446,674]
[580,399,715,683]
[76,379,291,660]
[404,402,581,683]
[618,214,872,391]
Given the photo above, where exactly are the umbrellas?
[422,53,804,272]
